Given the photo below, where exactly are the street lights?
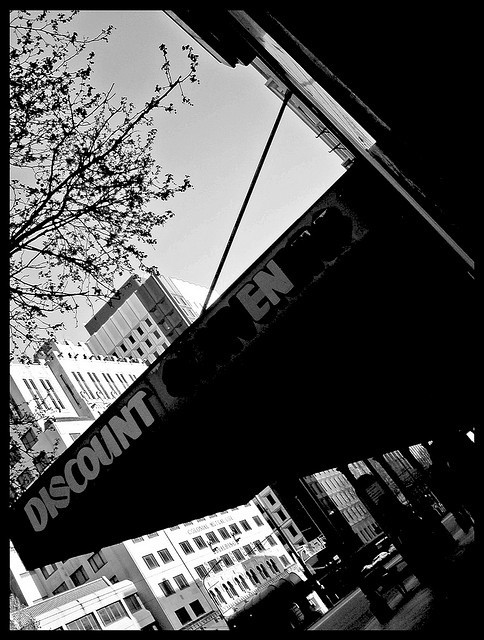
[201,557,232,630]
